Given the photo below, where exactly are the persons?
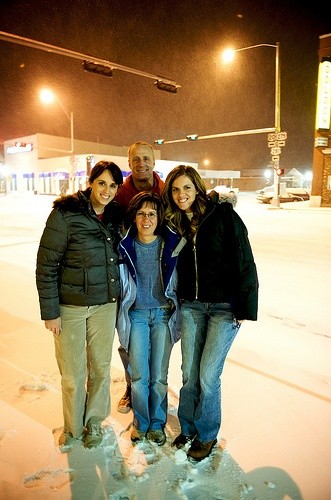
[161,164,259,464]
[36,161,123,451]
[115,142,187,446]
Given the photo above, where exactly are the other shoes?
[187,437,217,461]
[117,388,132,414]
[59,428,77,449]
[130,425,167,446]
[85,424,103,448]
[171,433,195,453]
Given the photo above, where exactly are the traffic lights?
[15,141,27,147]
[186,134,199,141]
[276,168,284,176]
[153,139,164,145]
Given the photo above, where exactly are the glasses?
[136,210,156,219]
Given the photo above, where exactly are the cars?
[256,186,310,204]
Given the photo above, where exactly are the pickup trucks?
[205,184,240,197]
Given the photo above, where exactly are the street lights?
[40,88,75,194]
[222,42,282,209]
